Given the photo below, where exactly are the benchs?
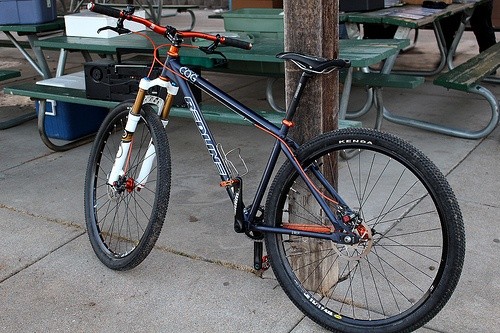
[0,3,500,162]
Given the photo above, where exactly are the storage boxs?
[221,8,284,41]
[35,71,111,141]
[0,0,57,25]
[64,10,146,39]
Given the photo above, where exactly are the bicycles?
[84,4,466,333]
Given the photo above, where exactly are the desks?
[0,18,92,80]
[339,0,480,120]
[33,30,411,120]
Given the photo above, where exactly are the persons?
[433,0,500,75]
